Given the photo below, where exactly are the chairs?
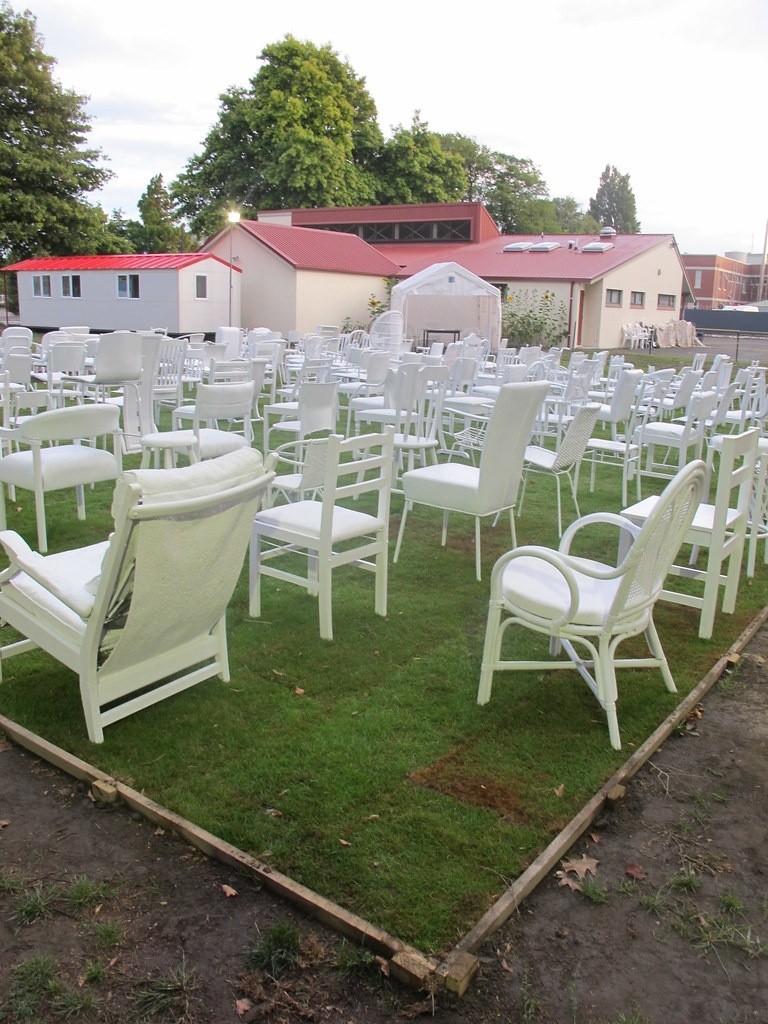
[0,310,768,752]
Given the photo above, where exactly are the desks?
[421,327,463,347]
